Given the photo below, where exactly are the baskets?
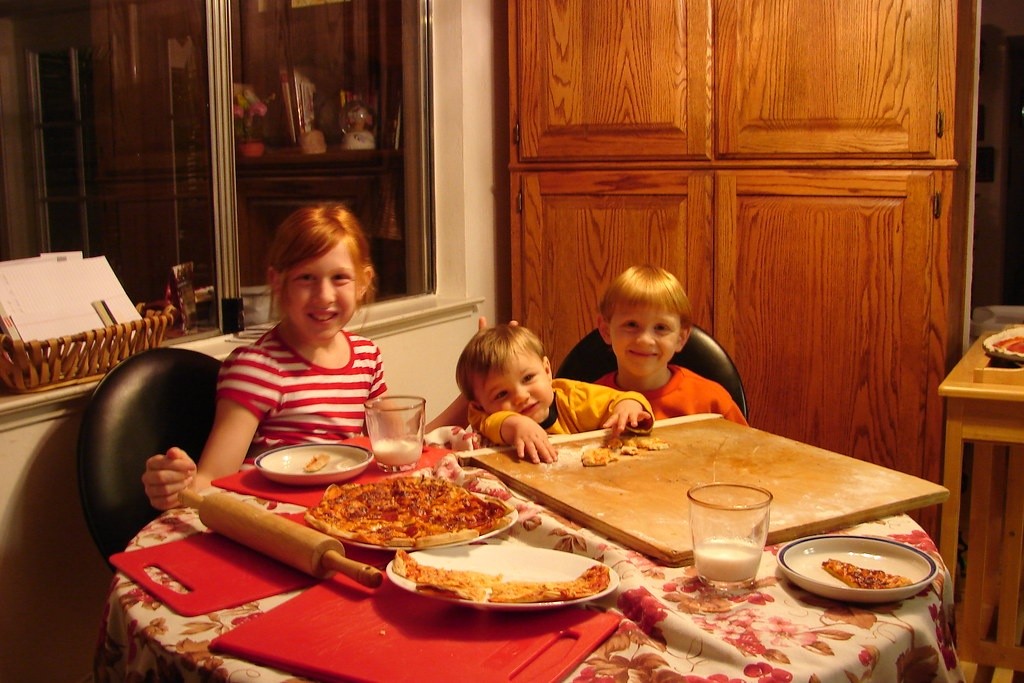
[0,303,177,395]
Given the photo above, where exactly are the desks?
[103,426,959,683]
[938,326,1024,673]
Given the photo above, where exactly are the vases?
[240,135,264,154]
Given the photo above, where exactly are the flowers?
[231,87,270,138]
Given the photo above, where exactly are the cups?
[686,481,773,594]
[365,395,427,471]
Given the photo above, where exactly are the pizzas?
[391,548,507,601]
[824,558,913,589]
[304,474,516,547]
[581,435,669,466]
[488,563,611,600]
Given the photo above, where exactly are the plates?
[385,544,621,611]
[255,444,375,484]
[776,534,939,601]
[982,326,1024,361]
[341,490,519,551]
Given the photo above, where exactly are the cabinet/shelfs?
[511,0,980,546]
[90,0,400,304]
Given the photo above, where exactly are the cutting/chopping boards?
[211,435,459,509]
[110,511,393,617]
[208,567,623,683]
[472,418,949,569]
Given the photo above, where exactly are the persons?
[456,324,654,464]
[592,265,748,425]
[142,205,519,508]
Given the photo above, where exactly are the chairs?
[556,324,751,428]
[76,347,230,578]
